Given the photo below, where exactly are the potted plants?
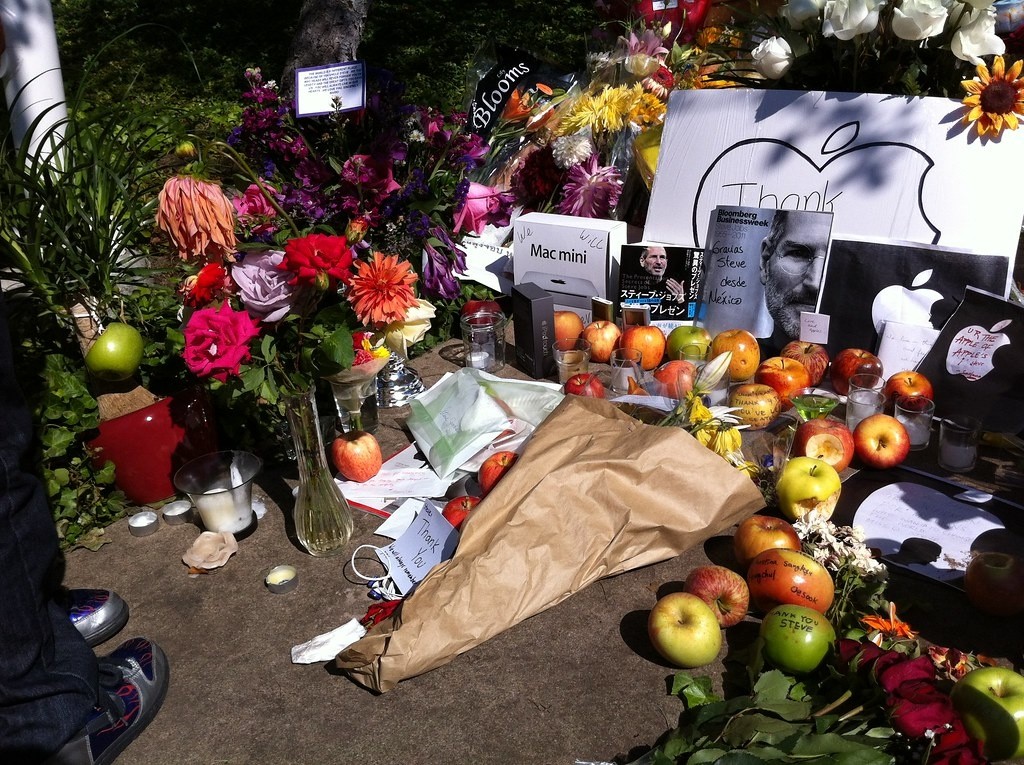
[0,21,219,505]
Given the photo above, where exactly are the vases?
[330,383,379,433]
[282,387,354,557]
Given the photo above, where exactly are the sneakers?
[45,635,170,765]
[67,587,129,649]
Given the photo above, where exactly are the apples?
[646,516,836,674]
[83,322,143,381]
[478,451,519,497]
[552,310,931,520]
[331,430,382,483]
[442,495,483,533]
[461,299,501,326]
[947,666,1024,761]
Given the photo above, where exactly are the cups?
[460,307,508,374]
[679,344,730,409]
[552,338,592,385]
[788,388,840,422]
[610,348,642,395]
[173,449,263,534]
[938,413,982,471]
[847,374,887,433]
[894,396,935,451]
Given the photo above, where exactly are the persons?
[0,241,170,765]
[640,248,687,322]
[758,211,834,364]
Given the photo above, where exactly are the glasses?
[773,241,825,276]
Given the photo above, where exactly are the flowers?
[0,0,1024,765]
